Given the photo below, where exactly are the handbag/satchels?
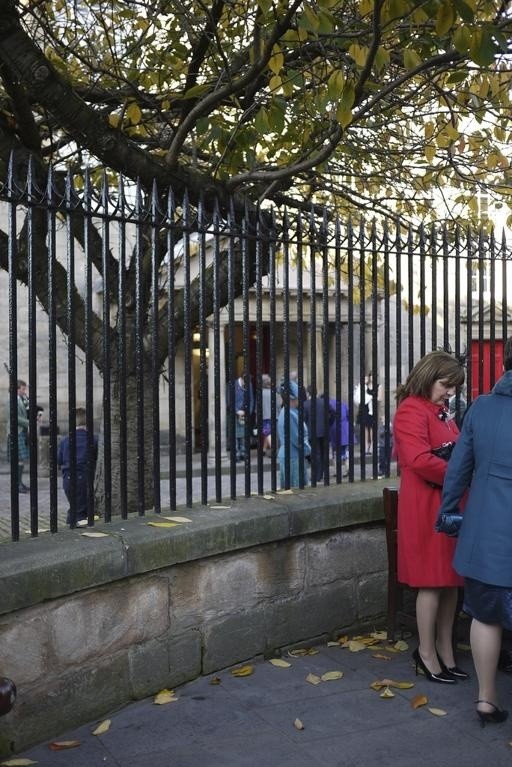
[436,514,463,532]
[428,441,456,487]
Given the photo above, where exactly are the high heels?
[413,648,468,683]
[474,701,508,728]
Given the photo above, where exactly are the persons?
[232,369,469,489]
[56,407,99,524]
[5,379,44,494]
[393,349,473,686]
[437,334,511,728]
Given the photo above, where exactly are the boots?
[18,464,30,493]
